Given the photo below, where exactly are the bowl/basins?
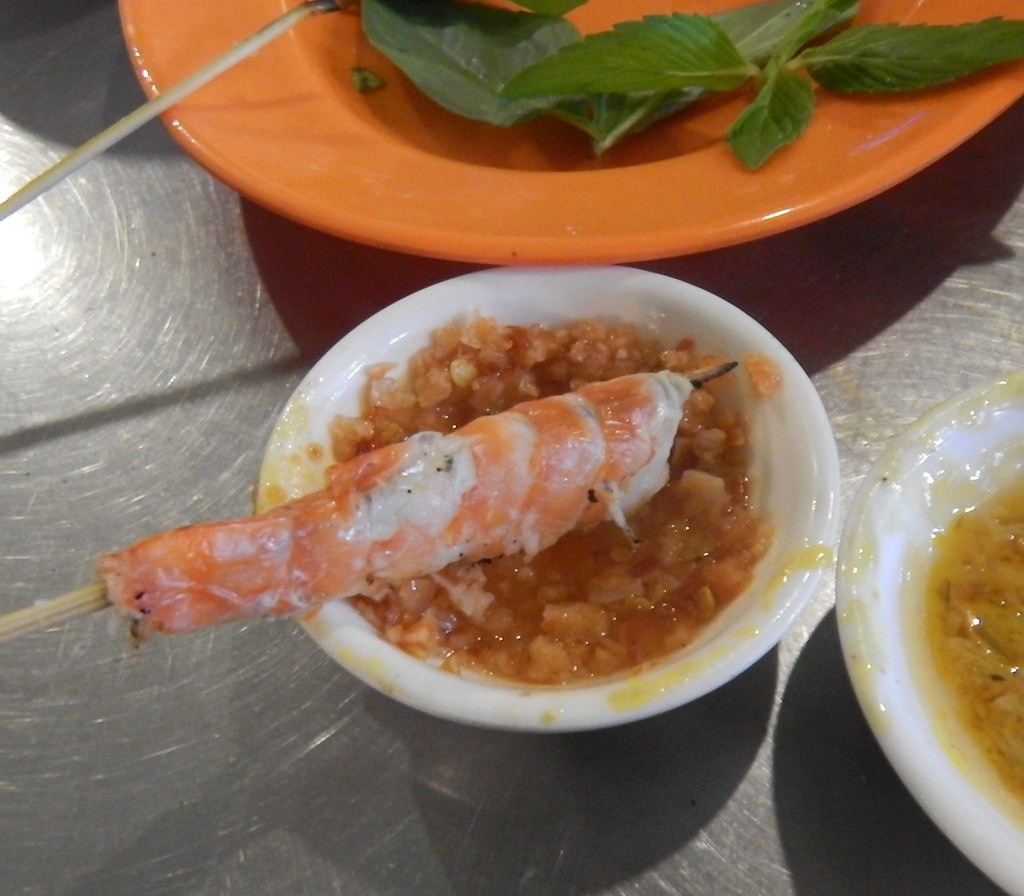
[260,264,839,733]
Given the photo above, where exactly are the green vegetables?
[359,0,1024,167]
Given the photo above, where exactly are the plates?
[116,0,1024,264]
[838,373,1024,896]
